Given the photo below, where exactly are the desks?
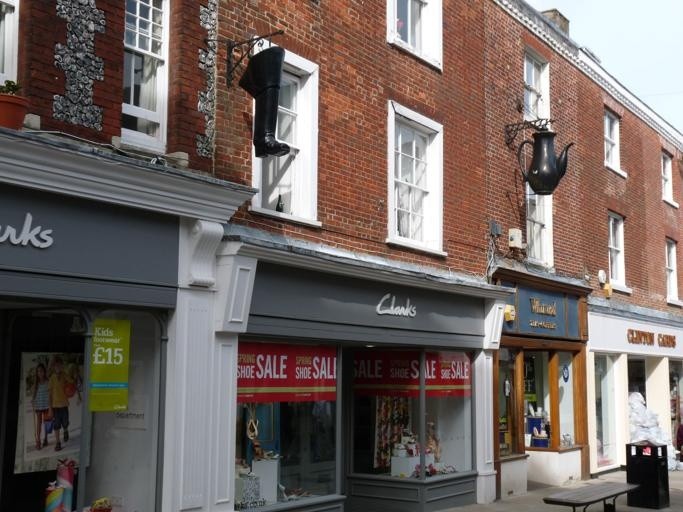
[543,481,642,512]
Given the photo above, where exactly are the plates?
[529,405,535,417]
[533,427,538,437]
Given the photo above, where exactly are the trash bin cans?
[626,440,670,509]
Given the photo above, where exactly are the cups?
[535,412,541,416]
[541,431,546,436]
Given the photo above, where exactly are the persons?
[45,357,75,450]
[29,361,49,449]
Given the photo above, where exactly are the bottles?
[393,432,417,458]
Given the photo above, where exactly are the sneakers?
[33,430,70,452]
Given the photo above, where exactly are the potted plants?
[1,79,33,130]
